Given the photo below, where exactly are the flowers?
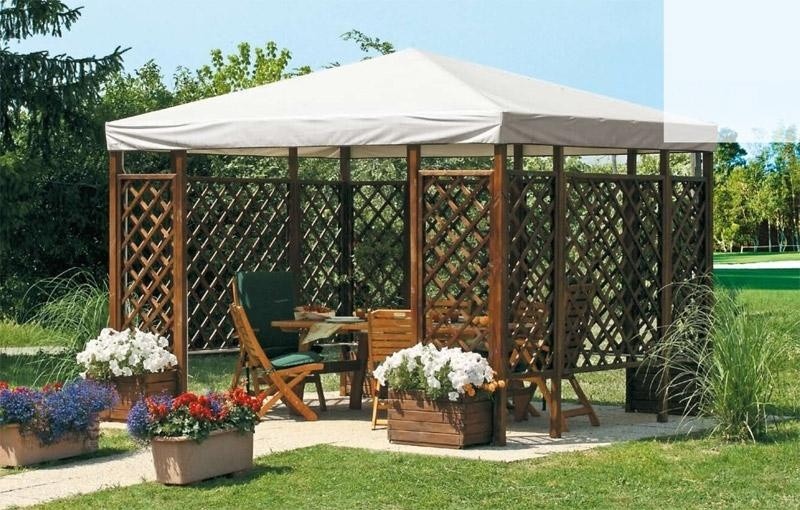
[128,387,267,442]
[0,376,120,444]
[77,326,177,380]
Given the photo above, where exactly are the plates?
[332,316,360,321]
[325,319,365,324]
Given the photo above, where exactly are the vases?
[89,370,176,423]
[387,388,492,449]
[150,425,254,483]
[0,413,100,469]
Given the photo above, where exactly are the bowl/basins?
[303,312,328,321]
